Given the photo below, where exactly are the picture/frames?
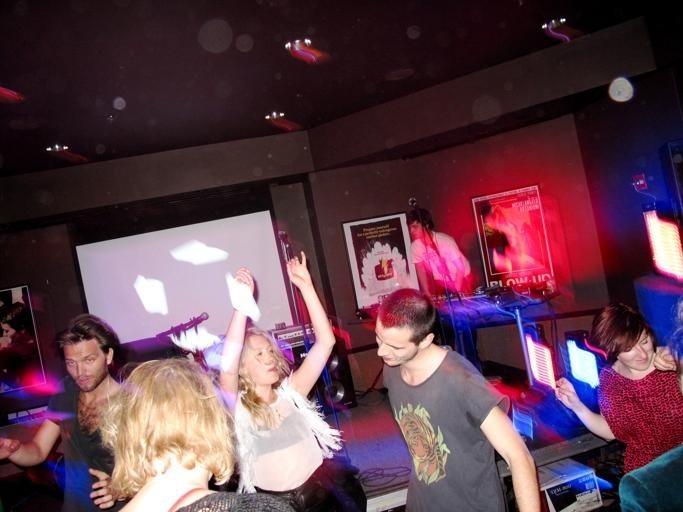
[340,211,423,311]
[472,185,554,289]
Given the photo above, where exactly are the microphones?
[408,197,417,207]
[278,231,290,248]
[185,312,208,329]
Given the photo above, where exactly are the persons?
[98,357,296,512]
[555,303,683,511]
[407,209,474,296]
[219,251,367,512]
[375,288,541,511]
[1,313,134,510]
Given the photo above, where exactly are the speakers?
[281,334,358,415]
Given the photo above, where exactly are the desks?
[434,304,607,376]
